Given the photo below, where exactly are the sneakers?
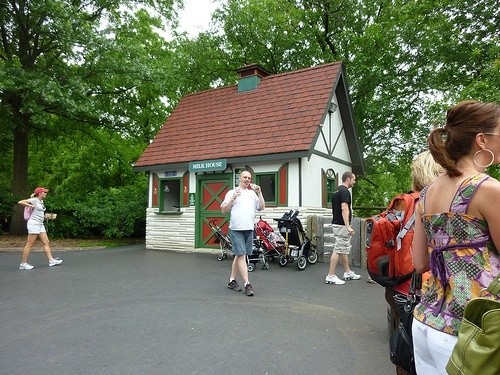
[228,280,242,292]
[245,283,255,297]
[325,274,345,285]
[343,272,361,280]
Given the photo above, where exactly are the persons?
[412,100,500,375]
[325,172,361,285]
[17,187,63,270]
[388,100,448,375]
[220,170,265,296]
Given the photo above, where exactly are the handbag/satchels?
[445,272,500,375]
[23,205,32,221]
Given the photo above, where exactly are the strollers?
[246,210,319,272]
[208,219,235,261]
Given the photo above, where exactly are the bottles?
[249,183,259,190]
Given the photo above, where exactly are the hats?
[34,187,49,195]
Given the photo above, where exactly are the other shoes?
[18,263,34,270]
[49,260,63,266]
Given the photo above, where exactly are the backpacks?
[364,190,422,289]
